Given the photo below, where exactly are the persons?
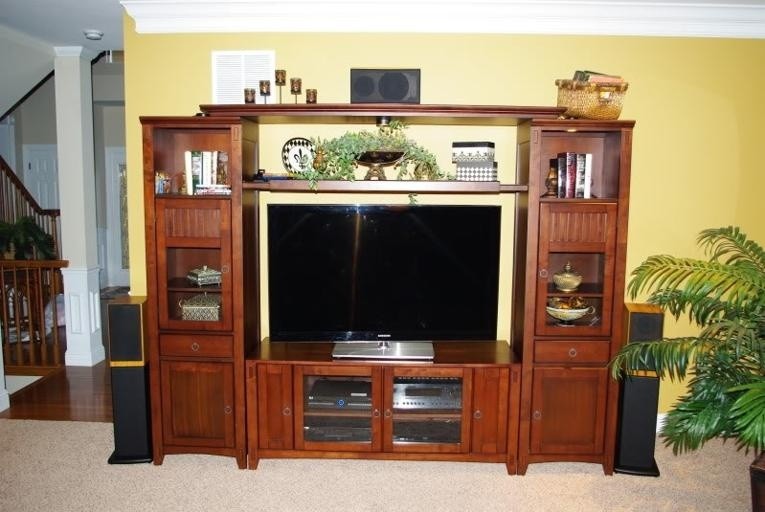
[44,290,67,350]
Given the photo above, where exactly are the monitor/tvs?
[267,202,503,360]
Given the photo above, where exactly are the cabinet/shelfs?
[137,115,259,473]
[201,105,569,192]
[246,339,517,470]
[518,117,637,478]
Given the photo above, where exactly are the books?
[184,150,232,196]
[572,71,623,82]
[557,152,592,199]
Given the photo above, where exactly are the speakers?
[106,295,153,464]
[349,67,421,104]
[614,301,661,478]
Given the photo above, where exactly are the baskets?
[555,77,629,120]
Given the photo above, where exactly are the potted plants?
[605,223,765,512]
